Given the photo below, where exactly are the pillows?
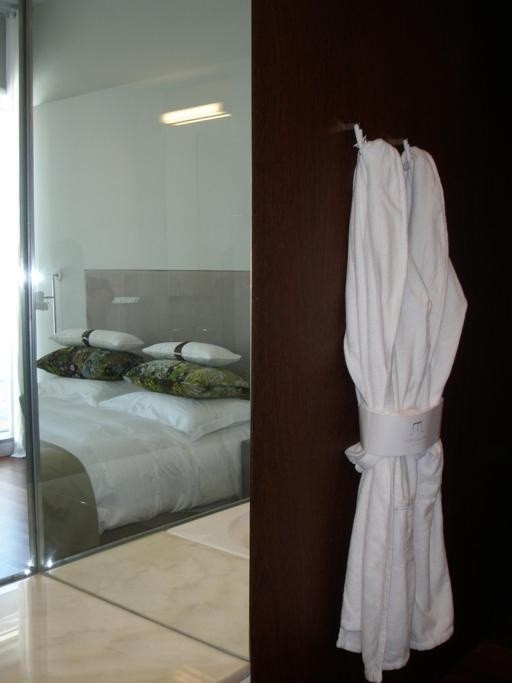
[37,344,148,382]
[126,358,252,399]
[100,390,251,438]
[140,340,247,371]
[46,327,145,352]
[37,374,141,412]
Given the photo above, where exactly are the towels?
[334,118,474,683]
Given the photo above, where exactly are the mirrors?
[18,0,253,666]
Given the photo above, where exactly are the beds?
[37,267,252,563]
